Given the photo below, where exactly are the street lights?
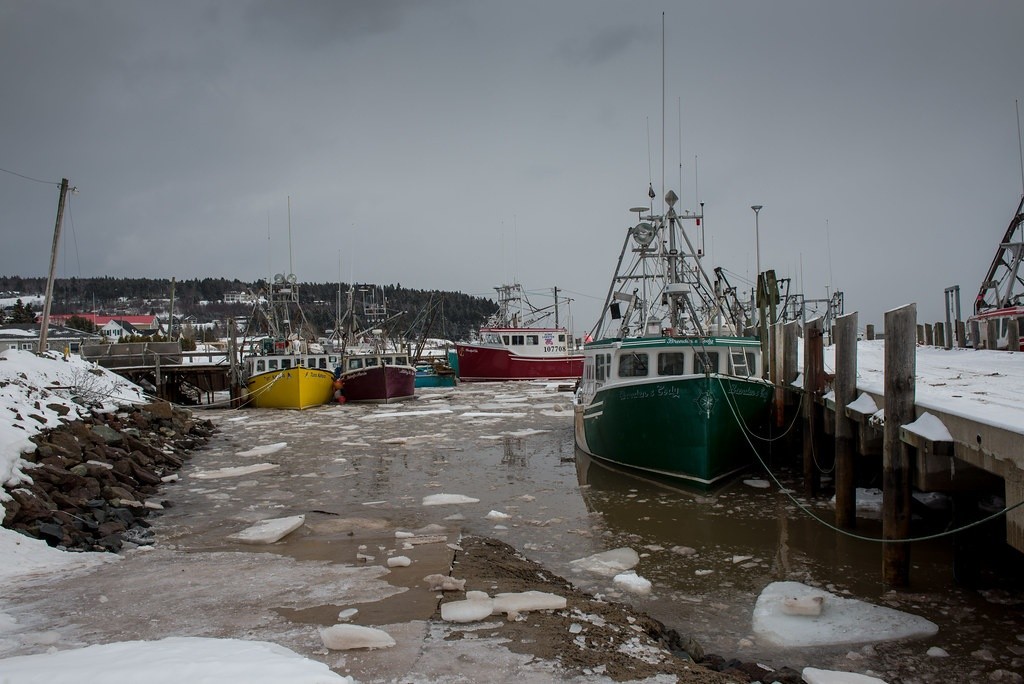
[37,178,79,357]
[751,206,764,276]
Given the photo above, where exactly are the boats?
[240,338,455,410]
[455,12,808,495]
